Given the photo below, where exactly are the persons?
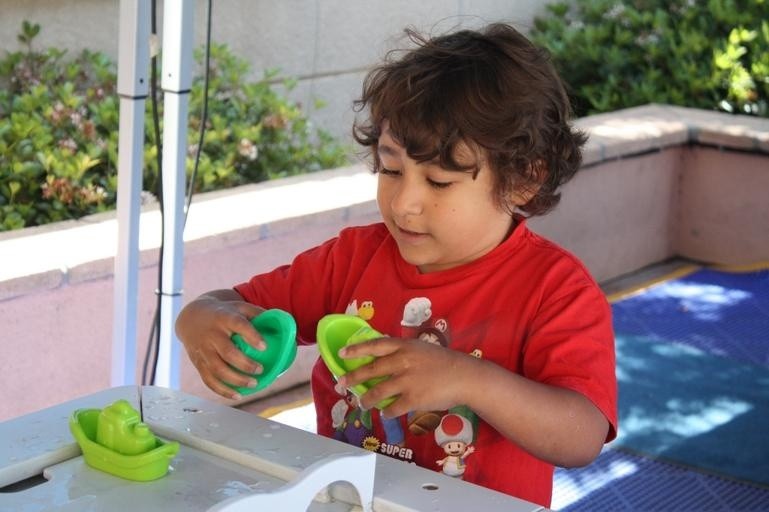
[173,22,619,509]
